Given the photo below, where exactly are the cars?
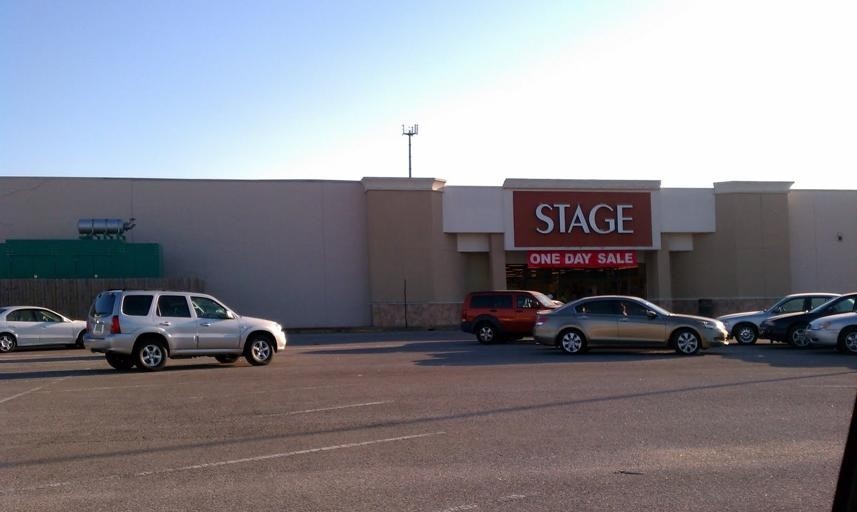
[532,294,730,356]
[714,292,857,354]
[0,305,89,351]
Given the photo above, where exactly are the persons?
[832,395,856,512]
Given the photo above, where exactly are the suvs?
[459,288,566,345]
[82,288,287,372]
